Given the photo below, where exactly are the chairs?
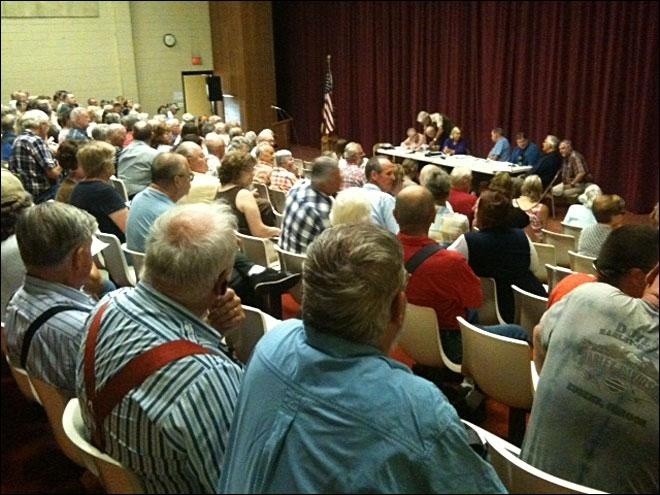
[370,142,395,161]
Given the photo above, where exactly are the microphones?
[425,152,441,156]
[270,104,282,110]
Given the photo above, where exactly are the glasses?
[174,173,194,181]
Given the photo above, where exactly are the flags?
[320,63,336,136]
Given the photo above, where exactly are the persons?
[0,88,660,495]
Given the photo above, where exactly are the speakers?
[206,76,222,101]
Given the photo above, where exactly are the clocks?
[163,33,176,47]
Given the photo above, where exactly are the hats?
[21,110,49,121]
[0,169,27,209]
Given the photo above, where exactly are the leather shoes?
[249,266,301,293]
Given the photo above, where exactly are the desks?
[376,144,534,197]
